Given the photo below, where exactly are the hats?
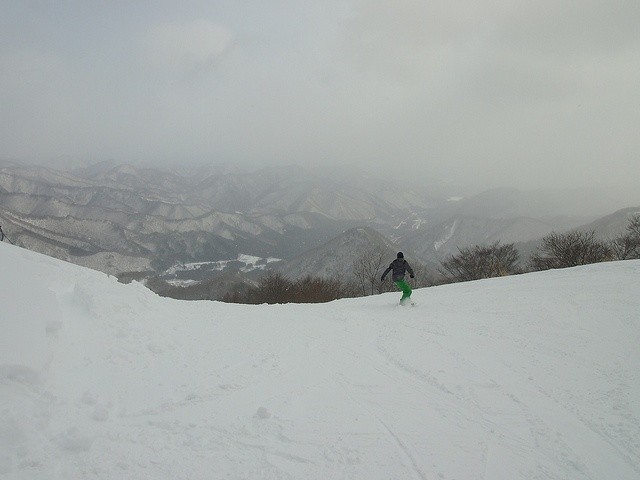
[397,252,403,258]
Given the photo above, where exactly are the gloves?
[381,275,385,281]
[410,274,414,278]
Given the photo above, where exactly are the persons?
[379,252,415,306]
